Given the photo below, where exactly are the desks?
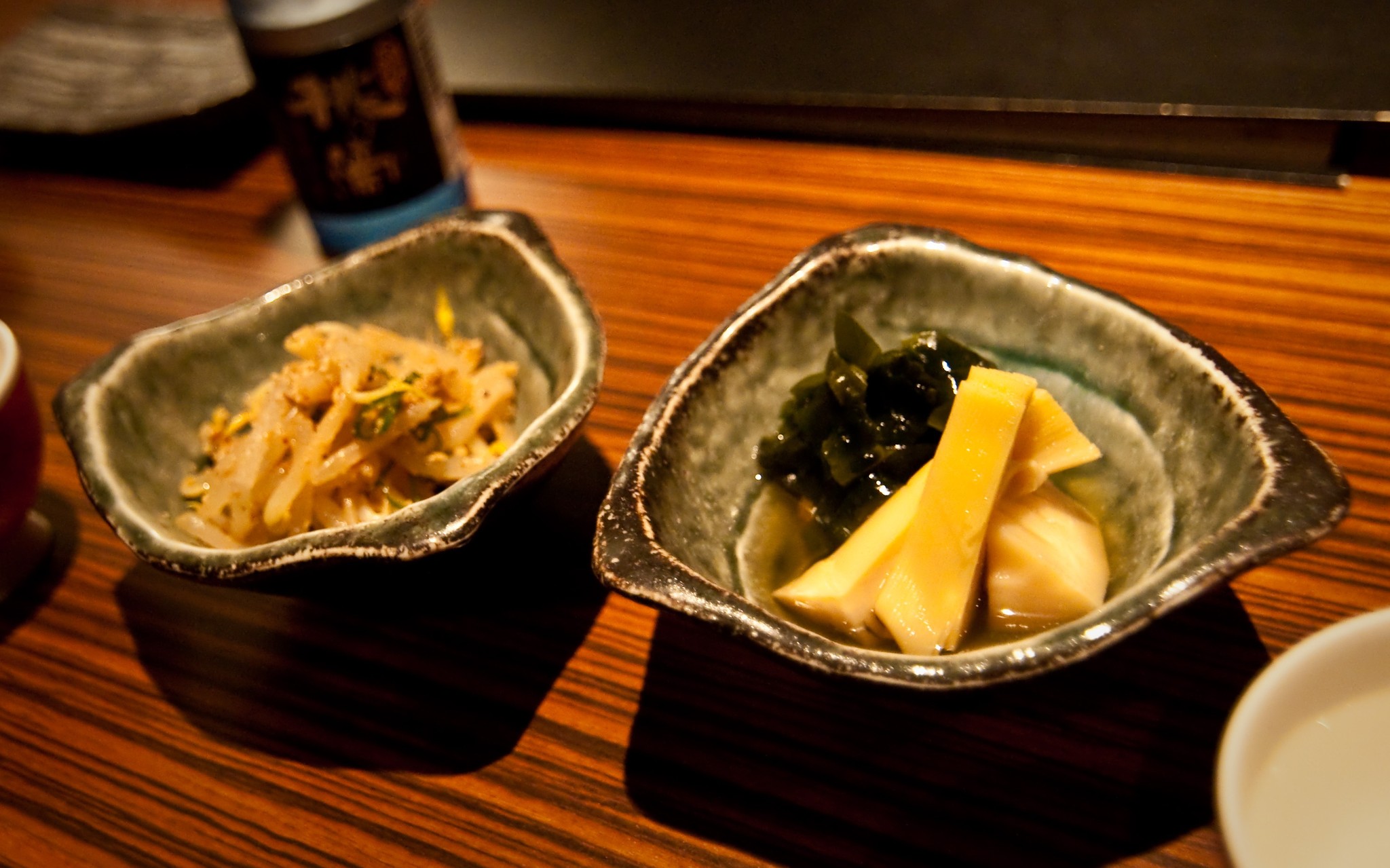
[0,108,1390,866]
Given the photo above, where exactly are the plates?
[587,221,1350,696]
[48,210,609,579]
[1216,610,1390,867]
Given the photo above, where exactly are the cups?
[0,318,56,606]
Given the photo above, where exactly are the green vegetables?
[356,362,473,510]
[754,316,997,532]
